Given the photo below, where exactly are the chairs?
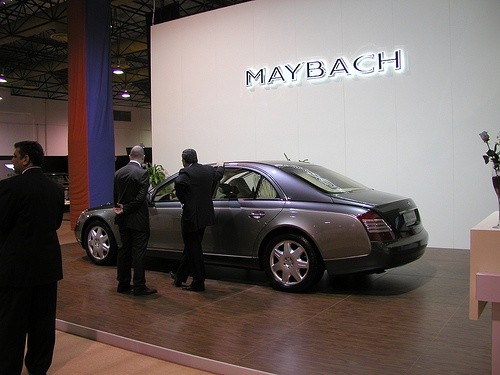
[229,177,251,198]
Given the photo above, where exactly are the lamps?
[112,58,124,75]
[121,77,130,97]
[0,65,7,83]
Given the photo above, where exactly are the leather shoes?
[170,272,182,287]
[183,284,204,291]
[117,284,133,293]
[133,287,158,296]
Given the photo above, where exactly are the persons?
[114,146,157,296]
[0,141,64,375]
[169,149,224,292]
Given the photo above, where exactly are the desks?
[469,210,500,321]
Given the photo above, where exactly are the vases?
[491,168,500,228]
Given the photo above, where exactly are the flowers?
[479,131,500,175]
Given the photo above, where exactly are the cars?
[72,158,430,294]
[46,172,70,212]
